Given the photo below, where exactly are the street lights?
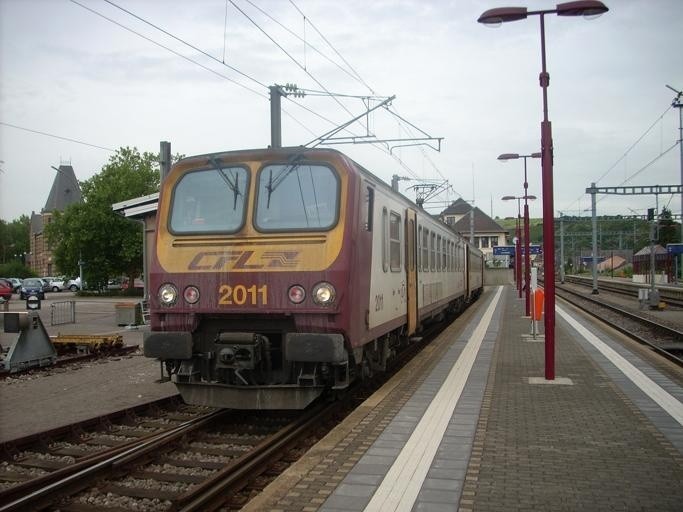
[500,195,538,299]
[496,152,542,317]
[476,0,610,380]
[51,165,84,291]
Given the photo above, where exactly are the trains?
[142,146,487,411]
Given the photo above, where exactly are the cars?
[0,275,87,300]
[108,277,143,288]
